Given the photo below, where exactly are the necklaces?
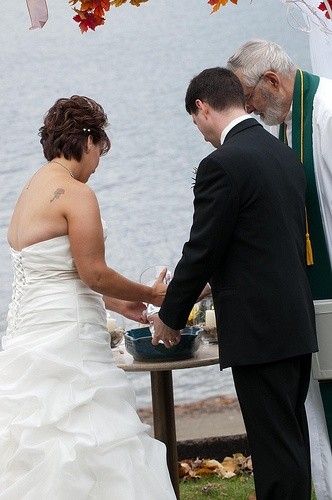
[47,161,76,178]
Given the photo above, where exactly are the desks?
[114,341,222,500]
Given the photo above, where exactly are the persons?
[0,95,178,499]
[145,67,318,500]
[225,39,331,453]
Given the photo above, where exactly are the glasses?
[243,73,267,112]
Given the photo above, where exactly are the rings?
[169,339,175,344]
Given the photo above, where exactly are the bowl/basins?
[124,325,204,361]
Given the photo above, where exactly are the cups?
[139,264,176,344]
